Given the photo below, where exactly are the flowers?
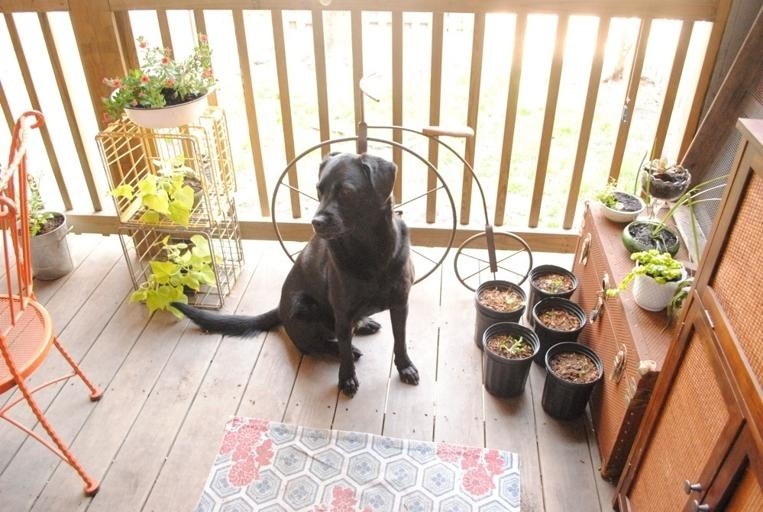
[92,28,217,115]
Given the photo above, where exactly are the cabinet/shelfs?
[606,116,763,512]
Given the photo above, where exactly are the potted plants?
[595,138,729,325]
[107,156,216,319]
[16,172,76,284]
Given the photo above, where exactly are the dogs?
[168,152,419,399]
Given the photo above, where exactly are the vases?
[472,264,604,422]
[108,84,216,130]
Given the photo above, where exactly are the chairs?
[0,107,110,496]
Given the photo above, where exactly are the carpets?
[192,416,525,512]
